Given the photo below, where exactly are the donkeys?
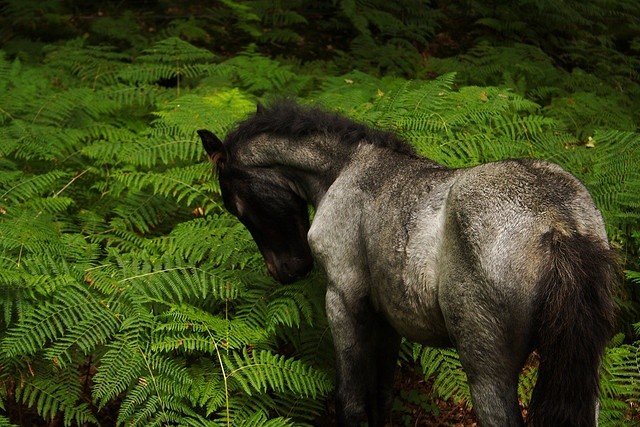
[196,99,626,427]
